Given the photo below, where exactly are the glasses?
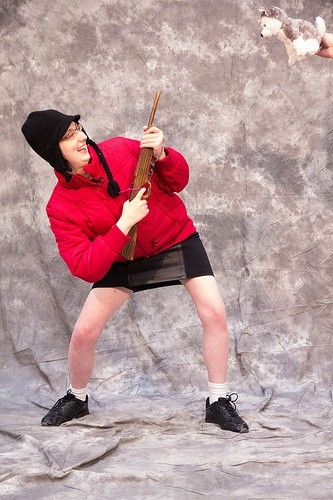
[58,123,84,142]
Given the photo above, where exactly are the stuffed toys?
[257,6,326,66]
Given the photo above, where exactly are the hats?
[21,109,120,199]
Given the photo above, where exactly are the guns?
[121,91,162,261]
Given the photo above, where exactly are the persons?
[315,32,333,59]
[21,109,249,433]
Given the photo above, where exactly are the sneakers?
[41,389,90,427]
[204,392,250,433]
